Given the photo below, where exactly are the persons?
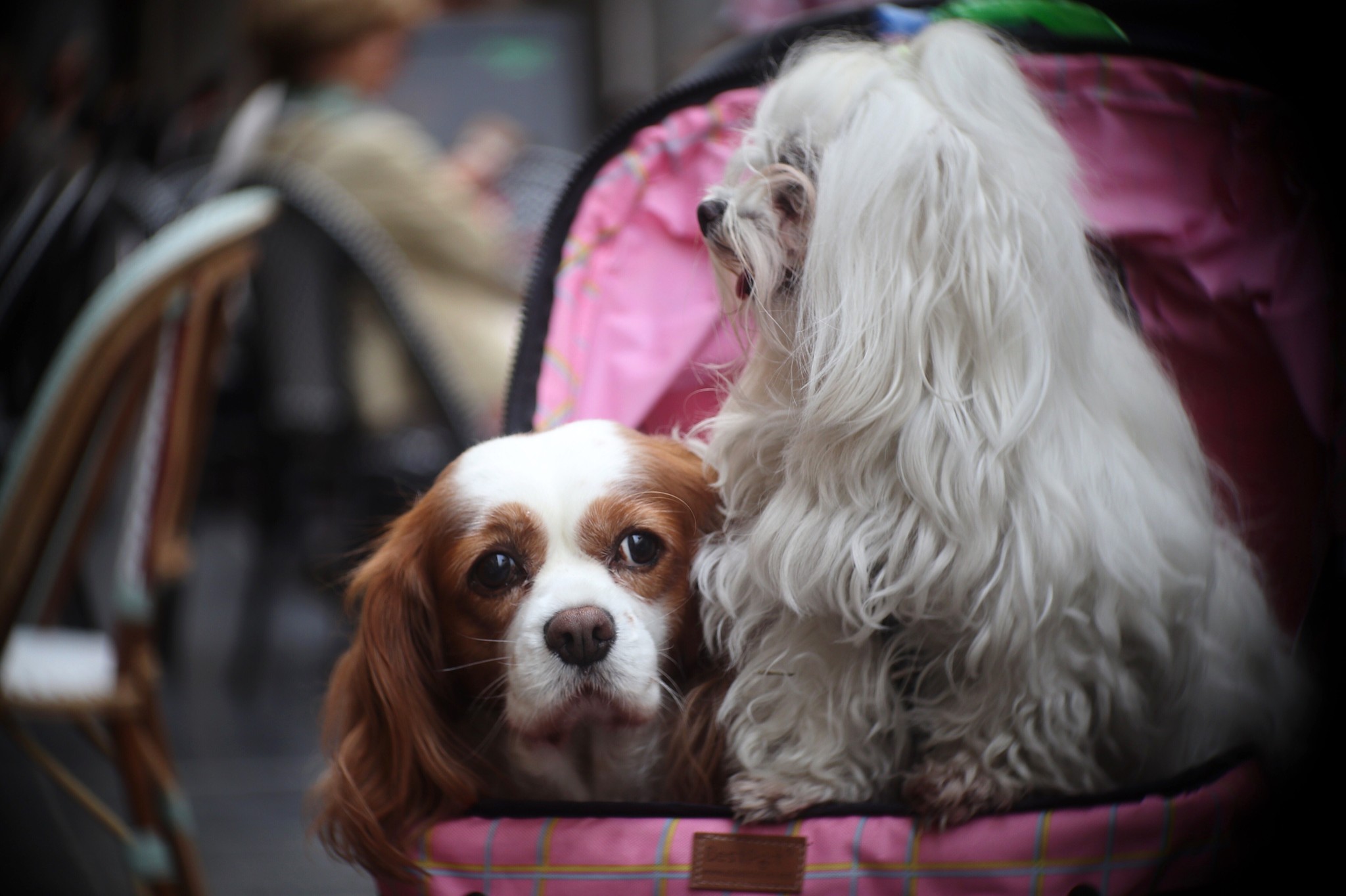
[215,1,545,442]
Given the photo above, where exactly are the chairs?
[0,159,502,896]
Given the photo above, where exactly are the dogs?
[670,14,1315,835]
[298,414,734,896]
